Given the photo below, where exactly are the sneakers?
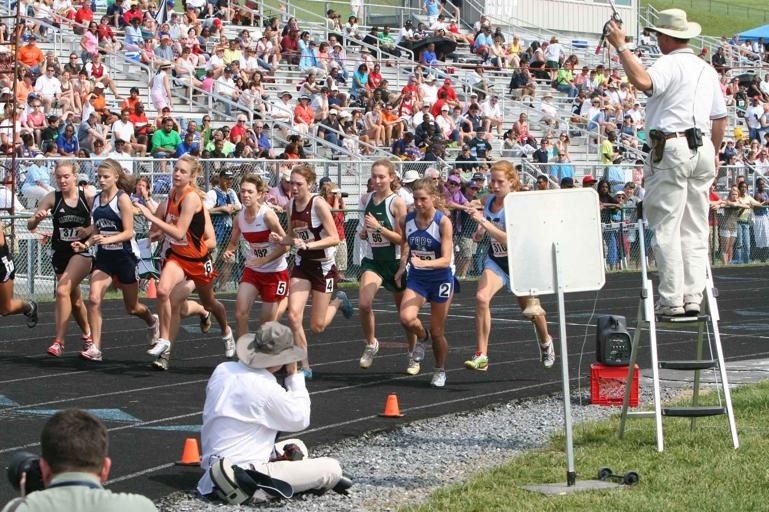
[199,311,213,334]
[146,314,160,345]
[151,354,170,371]
[429,367,447,387]
[46,338,64,357]
[80,334,94,351]
[358,337,379,368]
[412,327,432,362]
[145,338,171,357]
[24,299,39,329]
[539,335,556,369]
[654,302,685,317]
[683,302,702,315]
[79,344,104,362]
[332,290,354,319]
[406,356,422,375]
[297,367,313,381]
[220,325,235,359]
[463,351,489,372]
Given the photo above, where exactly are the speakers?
[596,314,633,366]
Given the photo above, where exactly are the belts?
[665,132,704,139]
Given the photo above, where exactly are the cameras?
[6,452,45,492]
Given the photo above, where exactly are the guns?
[602,0,622,37]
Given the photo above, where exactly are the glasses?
[48,69,55,72]
[115,143,124,146]
[150,6,156,8]
[471,96,478,99]
[496,40,499,42]
[601,186,609,188]
[79,152,85,155]
[204,119,210,122]
[33,105,40,107]
[238,120,246,122]
[256,126,263,128]
[66,129,73,132]
[17,107,25,111]
[432,177,440,180]
[616,196,625,199]
[386,108,393,111]
[449,181,459,187]
[224,130,230,133]
[542,142,548,145]
[140,106,144,108]
[70,57,78,60]
[163,111,169,113]
[94,145,103,147]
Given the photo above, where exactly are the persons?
[240,146,252,158]
[198,26,215,50]
[20,154,56,206]
[268,168,352,378]
[393,179,460,388]
[602,132,622,167]
[617,83,633,108]
[137,154,235,370]
[529,42,551,84]
[639,28,658,57]
[211,141,225,158]
[211,20,224,41]
[419,135,429,154]
[177,13,193,36]
[323,67,339,95]
[474,29,494,61]
[312,87,329,119]
[230,60,249,92]
[185,28,201,49]
[291,95,315,134]
[297,31,311,53]
[215,36,230,54]
[84,53,125,100]
[438,79,458,107]
[605,83,622,111]
[36,0,60,28]
[182,121,201,142]
[424,43,435,67]
[83,93,97,122]
[632,160,644,182]
[748,140,759,161]
[0,22,10,43]
[226,40,242,66]
[196,116,211,146]
[298,74,319,104]
[720,36,728,51]
[526,41,540,58]
[81,21,100,58]
[513,114,538,149]
[177,134,198,158]
[493,27,506,48]
[532,139,550,179]
[467,93,482,113]
[449,107,473,136]
[509,35,522,55]
[367,90,385,111]
[360,48,376,68]
[386,94,398,107]
[122,87,143,119]
[463,104,481,140]
[202,151,214,173]
[78,113,106,151]
[32,158,100,356]
[402,79,423,108]
[28,99,46,147]
[74,160,161,361]
[346,16,361,44]
[214,67,237,121]
[618,182,642,258]
[753,179,769,259]
[432,93,449,117]
[427,137,447,161]
[756,151,768,180]
[501,130,536,158]
[463,66,487,101]
[228,143,245,159]
[422,0,443,24]
[123,18,150,63]
[140,38,154,68]
[329,36,346,59]
[706,179,722,258]
[480,95,504,140]
[556,60,579,97]
[489,36,509,75]
[736,176,742,183]
[141,19,158,47]
[156,36,176,76]
[720,191,748,262]
[376,81,393,104]
[205,48,226,78]
[474,26,491,41]
[152,118,181,171]
[468,127,491,165]
[232,78,248,96]
[329,43,348,79]
[573,66,589,92]
[226,175,287,374]
[368,65,382,90]
[0,221,40,328]
[282,31,301,69]
[267,18,283,48]
[560,178,573,188]
[464,160,555,372]
[17,35,45,75]
[56,126,85,156]
[544,36,565,81]
[622,115,636,137]
[40,51,62,78]
[201,70,231,114]
[198,324,352,499]
[610,68,623,89]
[357,160,421,375]
[363,26,388,57]
[744,97,764,141]
[317,42,330,72]
[317,178,351,284]
[328,86,343,110]
[593,66,608,92]
[628,102,644,131]
[16,66,27,81]
[89,139,107,169]
[319,109,342,151]
[105,0,124,27]
[607,191,630,269]
[510,58,536,108]
[698,48,708,61]
[409,66,425,88]
[65,54,81,76]
[596,180,615,261]
[750,75,764,102]
[382,106,404,144]
[584,97,601,121]
[36,64,70,117]
[236,82,270,130]
[272,91,296,133]
[588,105,616,130]
[154,107,183,136]
[205,131,232,155]
[437,106,459,141]
[4,409,160,512]
[424,126,439,146]
[237,128,259,154]
[281,143,303,167]
[760,74,769,99]
[758,103,768,137]
[352,178,375,283]
[148,60,174,113]
[536,176,548,190]
[431,15,448,36]
[252,121,272,158]
[727,78,740,96]
[300,42,323,74]
[110,139,132,179]
[231,115,247,142]
[603,8,729,315]
[122,0,143,26]
[44,142,60,172]
[474,15,489,32]
[402,165,488,219]
[743,40,752,54]
[413,22,430,42]
[2,109,21,148]
[582,176,598,189]
[15,19,29,41]
[584,71,607,99]
[365,104,386,145]
[17,72,35,103]
[1,87,15,101]
[58,72,81,111]
[163,14,182,53]
[283,17,297,35]
[434,28,458,64]
[205,1,262,27]
[15,133,34,165]
[271,154,293,181]
[326,10,341,32]
[131,103,155,144]
[73,1,92,35]
[754,37,765,60]
[285,135,305,159]
[177,48,203,102]
[336,111,358,154]
[98,15,120,53]
[736,182,761,264]
[112,111,147,155]
[562,55,578,76]
[161,1,177,24]
[446,19,469,46]
[238,30,253,58]
[183,3,197,25]
[417,74,437,108]
[730,36,739,52]
[159,25,172,59]
[250,72,262,90]
[553,151,575,186]
[257,34,278,70]
[734,140,745,163]
[144,2,159,28]
[713,46,731,76]
[416,115,441,143]
[352,63,371,97]
[76,149,96,184]
[40,117,61,150]
[1,103,17,126]
[457,146,479,179]
[398,21,416,48]
[73,72,90,101]
[723,139,737,163]
[219,126,230,138]
[553,133,570,164]
[377,24,401,57]
[747,152,758,183]
[349,110,369,153]
[391,133,413,158]
[540,91,558,121]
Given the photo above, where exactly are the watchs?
[615,43,630,54]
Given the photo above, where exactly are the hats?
[616,191,625,195]
[752,95,759,102]
[94,82,106,89]
[89,111,98,118]
[328,108,338,116]
[440,105,449,112]
[129,87,139,95]
[444,79,451,85]
[235,319,306,369]
[92,52,100,58]
[339,111,352,118]
[560,177,575,187]
[29,34,36,39]
[402,170,421,183]
[280,170,291,181]
[220,169,235,179]
[162,105,171,112]
[29,93,38,98]
[466,180,480,188]
[278,91,293,100]
[333,43,342,50]
[471,172,486,181]
[422,101,431,107]
[155,60,175,69]
[231,464,294,507]
[298,93,311,103]
[470,93,478,97]
[582,176,597,184]
[225,67,233,73]
[359,46,371,53]
[643,8,703,40]
[161,35,169,39]
[448,174,461,185]
[1,86,14,96]
